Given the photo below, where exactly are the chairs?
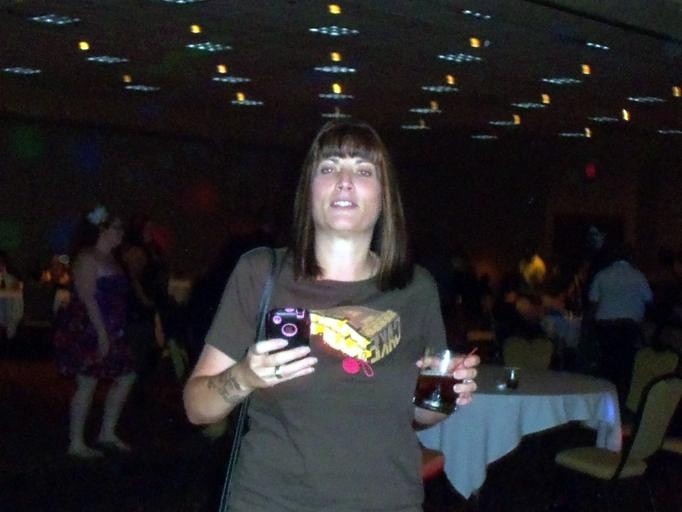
[551,373,680,507]
[500,334,556,371]
[598,344,681,511]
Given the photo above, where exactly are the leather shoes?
[97,435,131,452]
[67,445,102,458]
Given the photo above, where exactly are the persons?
[430,222,653,377]
[181,115,483,512]
[50,199,280,458]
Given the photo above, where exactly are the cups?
[505,366,521,391]
[412,344,467,416]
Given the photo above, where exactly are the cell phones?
[265,308,311,353]
